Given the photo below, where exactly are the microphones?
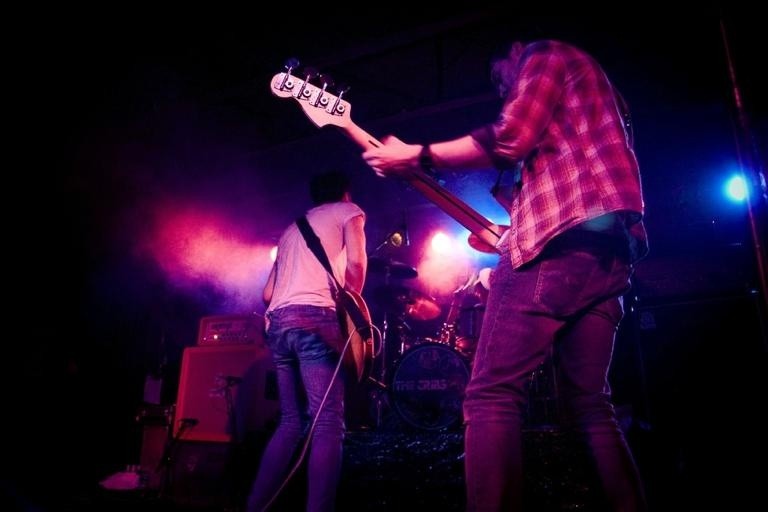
[179,418,197,426]
[402,212,411,247]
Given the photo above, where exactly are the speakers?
[172,346,259,442]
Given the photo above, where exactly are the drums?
[389,338,470,432]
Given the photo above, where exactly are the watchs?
[423,143,431,174]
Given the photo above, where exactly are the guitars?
[337,285,374,383]
[270,58,522,254]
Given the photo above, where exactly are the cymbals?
[368,256,418,280]
[375,285,443,320]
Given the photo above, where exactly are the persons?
[248,170,368,512]
[361,39,649,511]
